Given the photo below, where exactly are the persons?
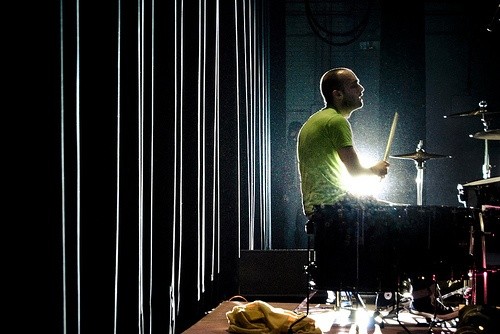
[283,121,308,248]
[297,67,459,320]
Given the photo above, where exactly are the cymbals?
[469,130,500,141]
[442,110,499,119]
[461,176,500,189]
[388,152,452,161]
